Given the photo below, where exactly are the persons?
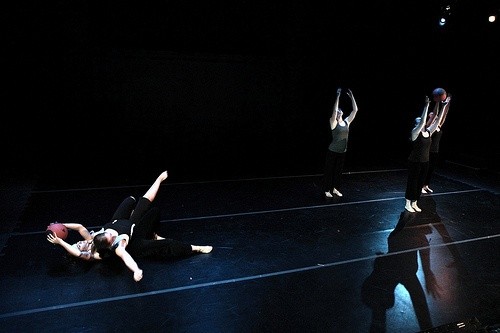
[421,97,451,193]
[46,170,213,283]
[404,95,448,213]
[323,89,358,197]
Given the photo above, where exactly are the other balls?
[46,224,68,239]
[433,88,447,100]
